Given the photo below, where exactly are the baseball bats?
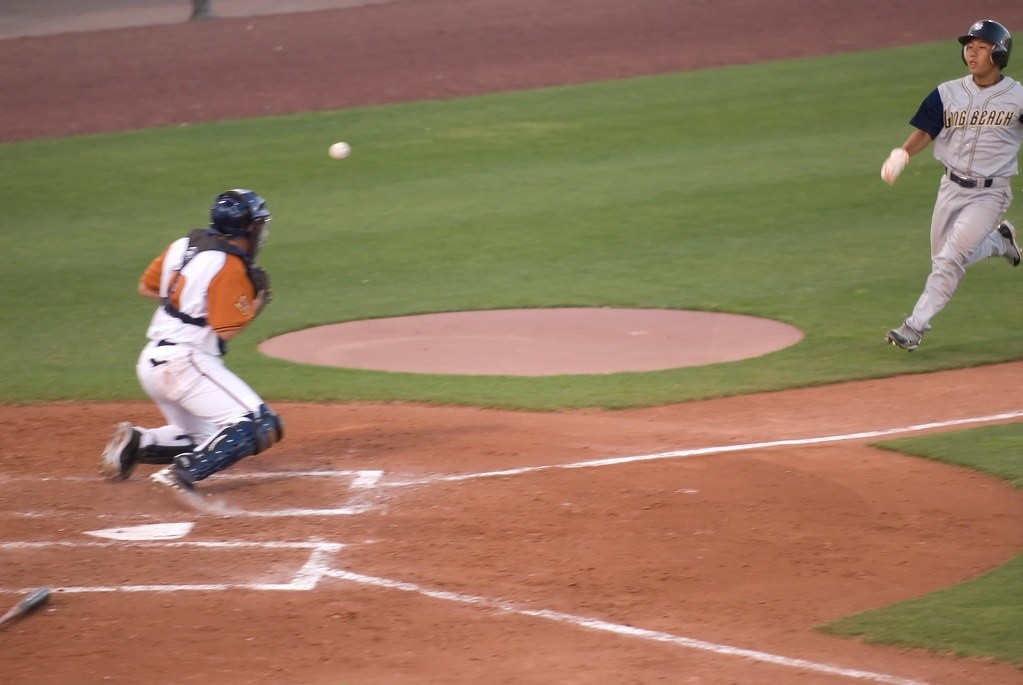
[0,588,49,625]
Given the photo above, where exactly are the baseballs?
[328,141,352,161]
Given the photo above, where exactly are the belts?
[157,340,175,346]
[945,166,993,188]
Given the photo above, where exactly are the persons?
[881,20,1023,353]
[100,188,284,496]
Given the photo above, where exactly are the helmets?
[212,185,270,235]
[959,20,1012,68]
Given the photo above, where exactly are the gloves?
[881,148,909,184]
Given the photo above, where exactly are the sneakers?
[996,220,1023,267]
[101,422,141,481]
[152,466,200,502]
[885,321,923,352]
